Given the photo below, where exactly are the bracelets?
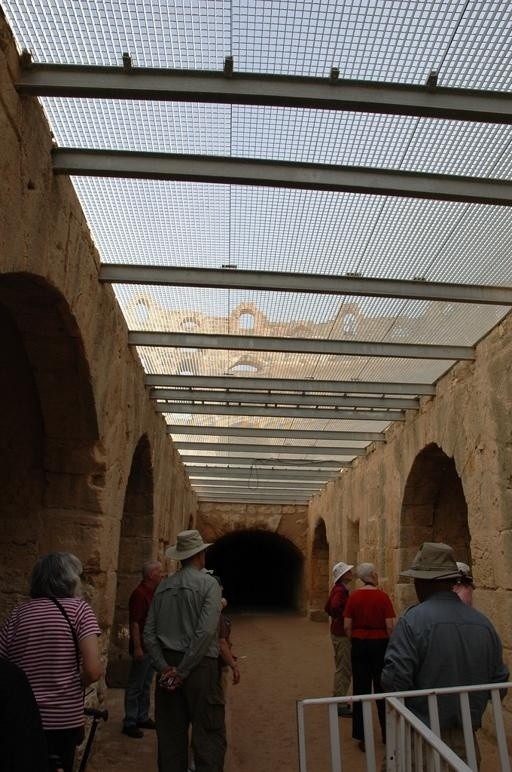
[232,662,237,670]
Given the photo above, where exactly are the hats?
[398,542,471,580]
[201,567,215,574]
[166,529,215,560]
[331,561,355,583]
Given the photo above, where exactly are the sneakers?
[338,708,353,717]
[122,717,157,738]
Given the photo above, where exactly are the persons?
[0,547,104,772]
[323,561,355,717]
[189,566,239,750]
[141,528,232,771]
[343,561,397,752]
[379,540,509,772]
[451,560,482,611]
[118,558,165,741]
